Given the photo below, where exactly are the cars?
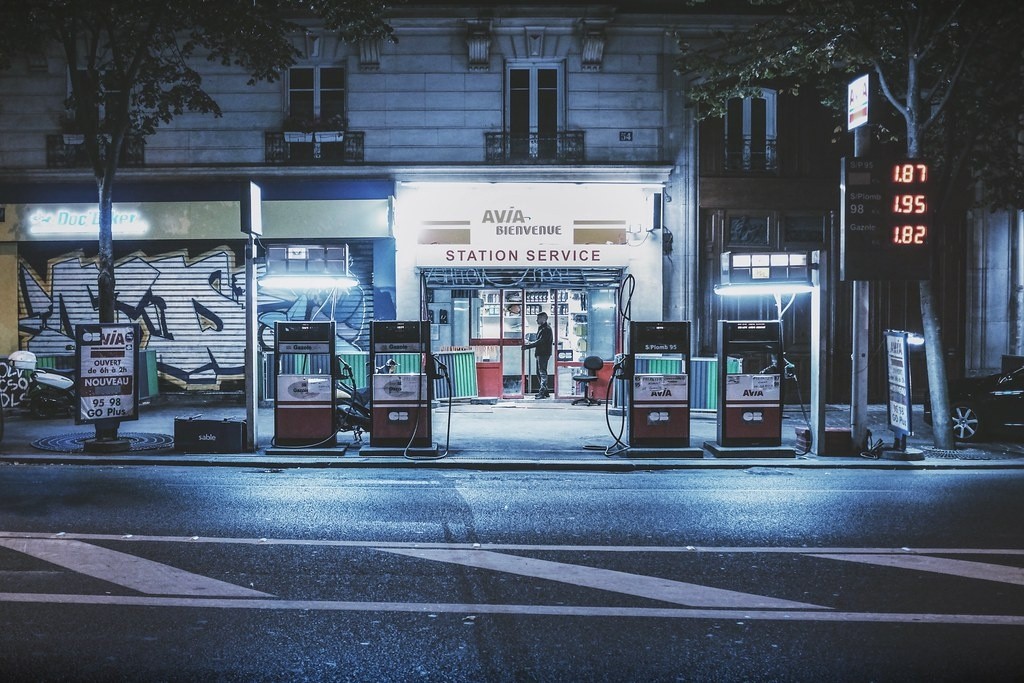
[923,366,1024,442]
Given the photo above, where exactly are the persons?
[521,312,553,399]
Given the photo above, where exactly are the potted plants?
[60,114,112,143]
[281,111,349,143]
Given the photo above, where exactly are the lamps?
[665,188,672,201]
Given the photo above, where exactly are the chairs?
[572,355,603,406]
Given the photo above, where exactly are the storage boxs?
[7,351,38,370]
[173,414,247,453]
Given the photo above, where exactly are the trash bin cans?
[139,350,161,401]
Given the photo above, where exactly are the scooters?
[7,344,76,418]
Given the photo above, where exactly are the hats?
[386,358,400,366]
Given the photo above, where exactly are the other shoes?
[535,392,550,399]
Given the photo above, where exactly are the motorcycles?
[334,359,400,443]
